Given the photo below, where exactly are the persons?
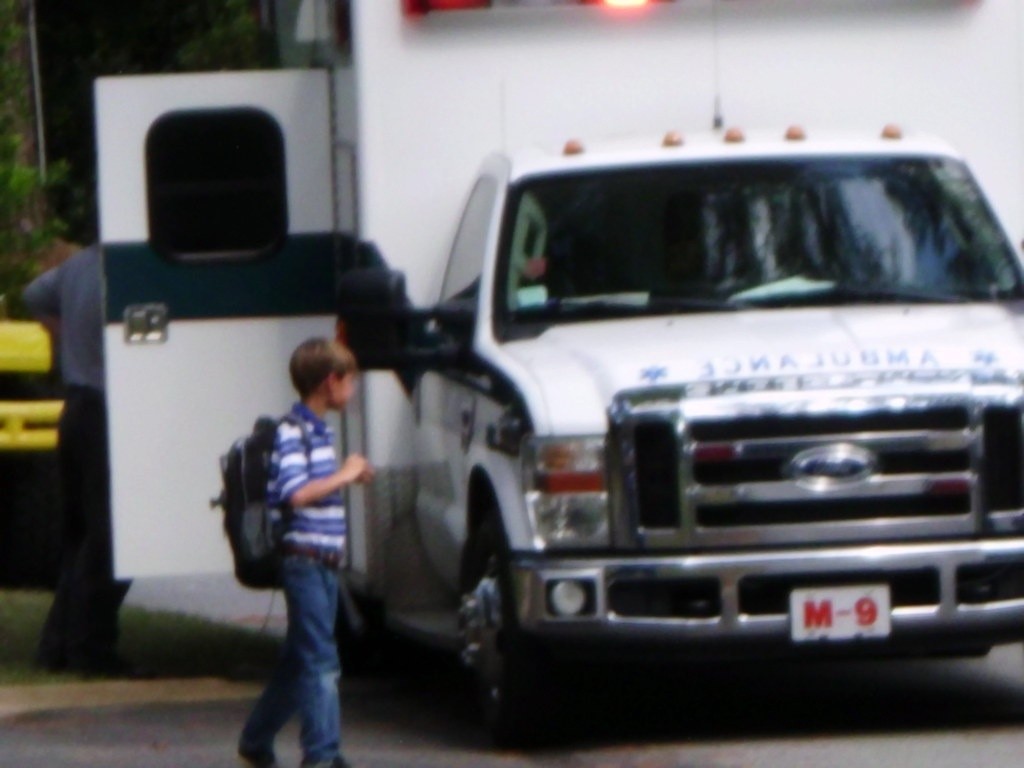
[234,338,374,768]
[21,240,133,676]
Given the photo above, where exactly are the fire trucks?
[92,0,1023,745]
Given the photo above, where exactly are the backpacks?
[211,416,313,590]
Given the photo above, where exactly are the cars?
[0,307,69,457]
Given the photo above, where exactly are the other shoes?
[234,739,280,768]
[300,755,351,768]
[33,640,154,679]
